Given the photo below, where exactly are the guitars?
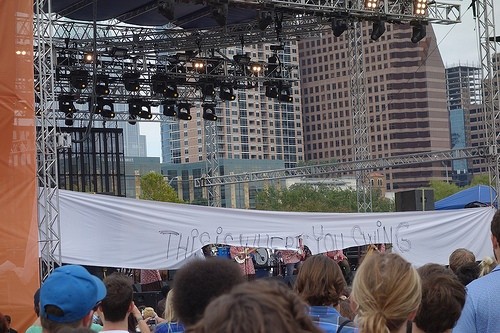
[234,247,259,264]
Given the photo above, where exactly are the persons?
[447,209,500,333]
[229,246,257,283]
[0,247,497,333]
[280,243,306,276]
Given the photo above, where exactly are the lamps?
[35,0,427,126]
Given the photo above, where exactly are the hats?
[40,265,108,323]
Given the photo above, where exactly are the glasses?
[92,301,103,311]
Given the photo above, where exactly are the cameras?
[149,319,156,324]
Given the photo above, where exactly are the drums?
[254,248,268,265]
